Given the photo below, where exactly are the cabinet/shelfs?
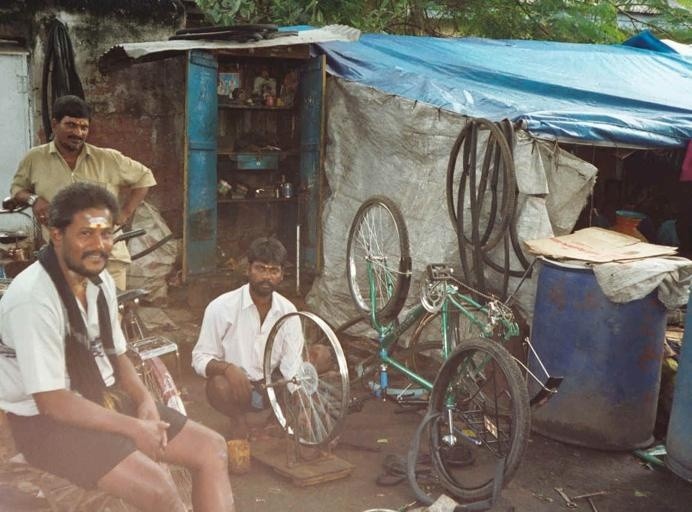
[182,49,326,286]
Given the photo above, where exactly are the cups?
[226,439,252,475]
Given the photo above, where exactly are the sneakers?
[228,436,250,473]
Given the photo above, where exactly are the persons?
[8,94,157,327]
[0,183,236,512]
[191,235,332,476]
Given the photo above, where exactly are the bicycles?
[345,196,520,459]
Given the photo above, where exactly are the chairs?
[0,412,193,511]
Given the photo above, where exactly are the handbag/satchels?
[66,335,136,417]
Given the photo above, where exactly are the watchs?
[26,193,39,207]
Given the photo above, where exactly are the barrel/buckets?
[523,258,665,451]
[665,291,692,484]
